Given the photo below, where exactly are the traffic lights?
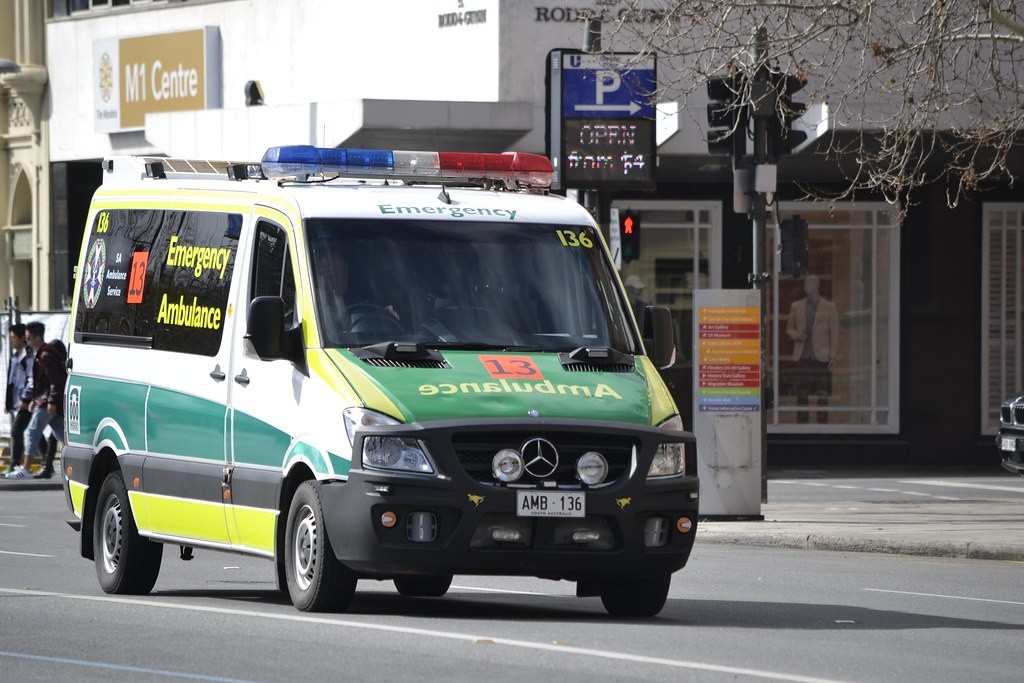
[620,207,643,264]
[703,63,750,169]
[769,64,807,159]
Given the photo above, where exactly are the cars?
[997,393,1024,479]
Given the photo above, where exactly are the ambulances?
[63,139,700,623]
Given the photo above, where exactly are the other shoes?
[0,469,14,479]
[32,471,51,479]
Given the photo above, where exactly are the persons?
[786,273,841,425]
[623,275,655,358]
[2,320,72,485]
[309,253,401,336]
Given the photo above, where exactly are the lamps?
[244,81,261,106]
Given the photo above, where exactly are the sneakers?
[5,465,33,480]
